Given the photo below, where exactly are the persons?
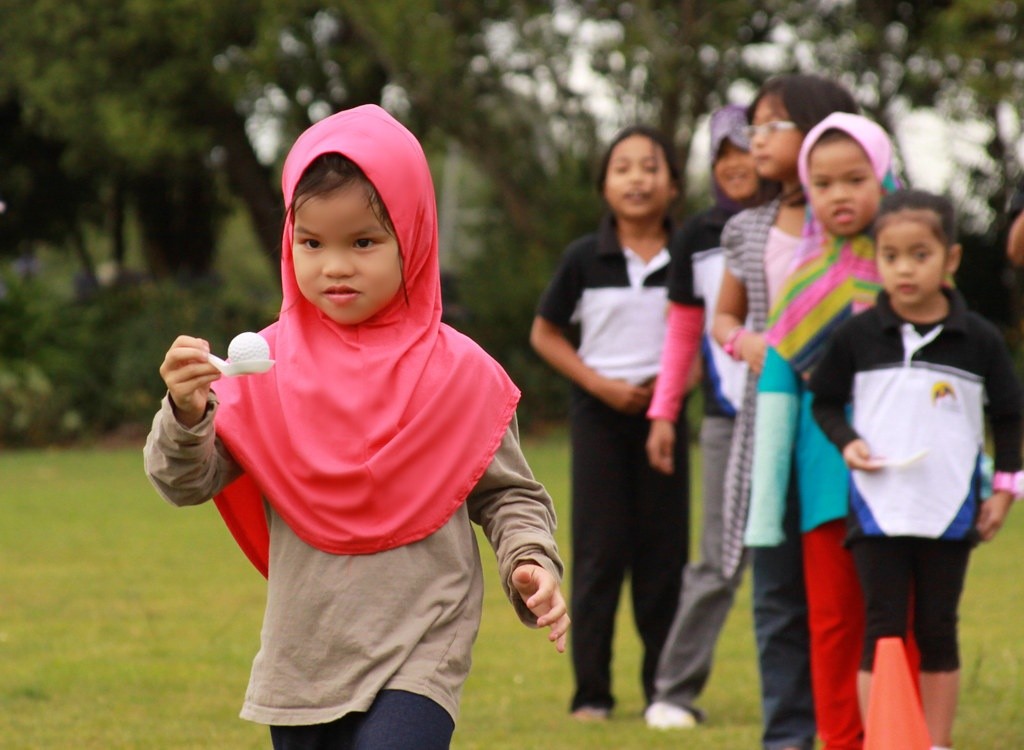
[808,190,1023,750]
[644,106,758,725]
[713,75,854,749]
[745,113,954,750]
[529,125,704,721]
[142,104,572,750]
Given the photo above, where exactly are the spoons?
[868,446,933,470]
[208,353,275,376]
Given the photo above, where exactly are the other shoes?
[645,700,694,734]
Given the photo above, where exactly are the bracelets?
[993,472,1023,501]
[725,327,746,359]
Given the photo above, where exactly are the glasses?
[743,120,808,141]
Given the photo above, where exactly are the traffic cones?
[864,637,932,750]
